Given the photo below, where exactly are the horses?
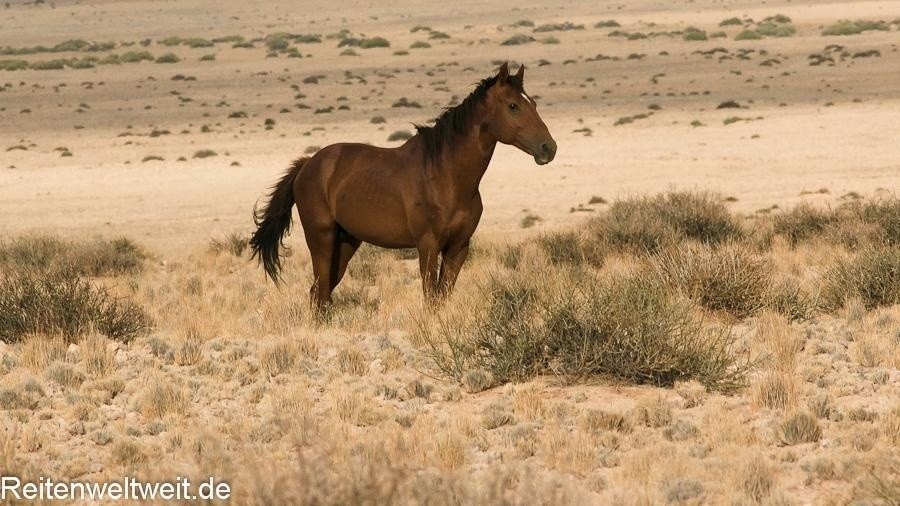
[248,60,557,325]
